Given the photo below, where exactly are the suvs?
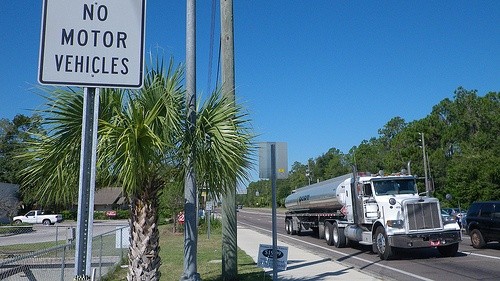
[466,200,500,248]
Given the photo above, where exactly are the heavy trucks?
[285,131,463,260]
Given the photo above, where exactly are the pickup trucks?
[12,209,61,226]
